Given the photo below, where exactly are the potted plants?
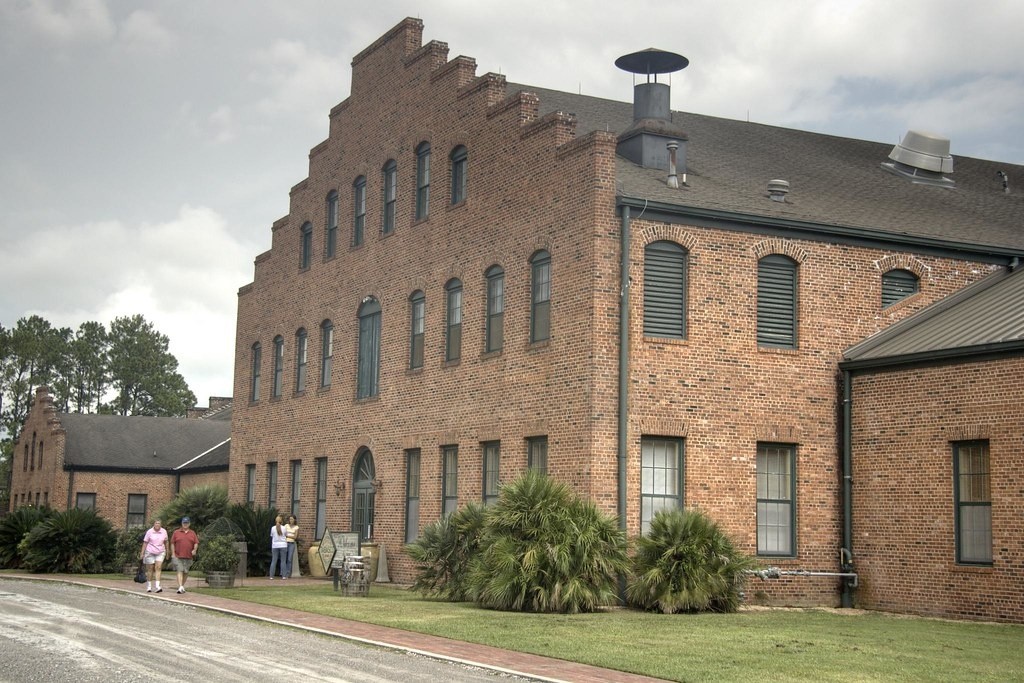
[195,535,241,589]
[116,525,144,574]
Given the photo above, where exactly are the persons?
[170,517,199,594]
[270,514,300,579]
[140,518,169,593]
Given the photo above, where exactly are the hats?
[182,517,190,523]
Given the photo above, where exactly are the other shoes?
[155,587,162,593]
[270,577,273,579]
[146,586,152,592]
[176,587,185,593]
[282,576,286,580]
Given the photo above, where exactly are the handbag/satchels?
[134,559,147,583]
